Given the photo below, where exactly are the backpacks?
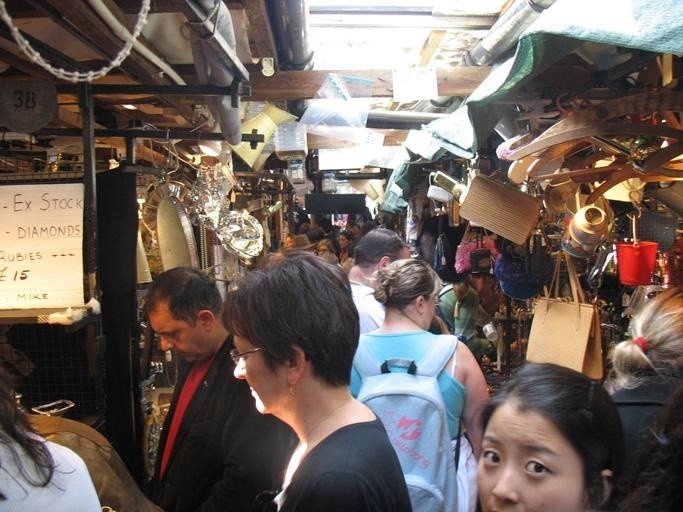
[351,334,459,511]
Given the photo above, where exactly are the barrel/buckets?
[616,215,659,286]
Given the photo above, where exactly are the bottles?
[138,335,173,386]
[483,323,498,343]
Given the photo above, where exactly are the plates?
[543,176,578,216]
[0,67,58,132]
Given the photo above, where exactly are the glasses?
[229,345,262,365]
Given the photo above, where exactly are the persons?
[1,218,682,511]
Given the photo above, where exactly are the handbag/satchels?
[454,230,502,275]
[494,243,554,301]
[525,251,603,380]
[433,234,454,273]
